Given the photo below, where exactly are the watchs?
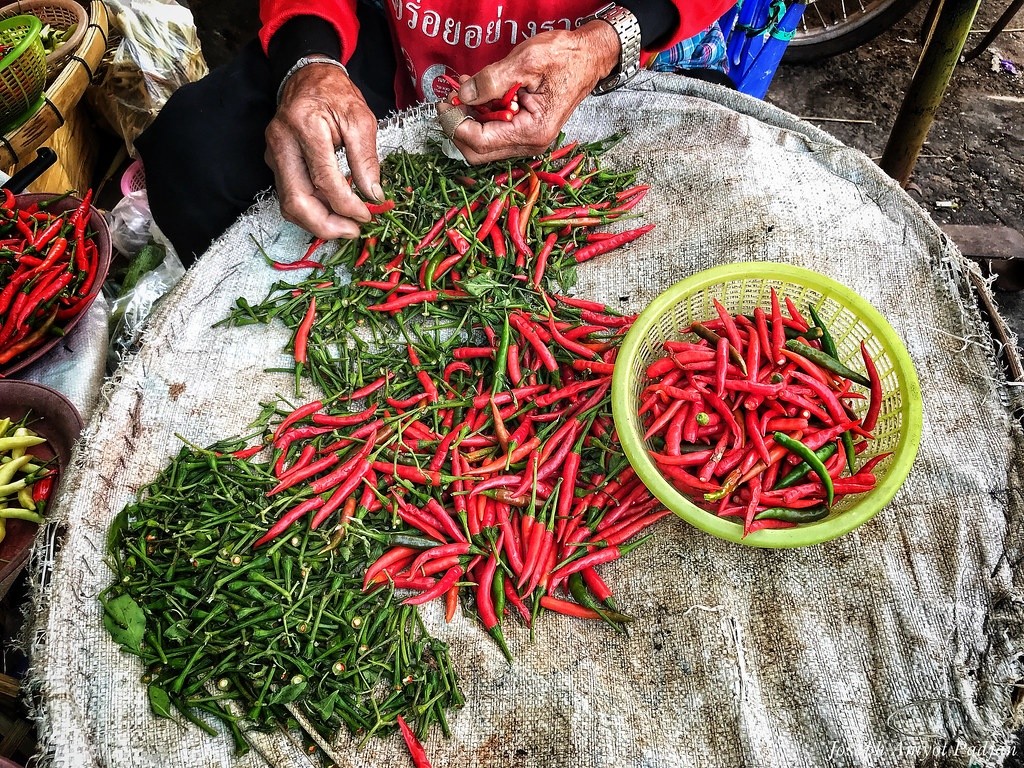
[580,1,641,98]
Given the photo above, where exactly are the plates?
[0,193,112,376]
[1,378,83,606]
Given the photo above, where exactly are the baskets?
[611,258,925,549]
[121,160,149,200]
[0,15,48,135]
[0,0,88,81]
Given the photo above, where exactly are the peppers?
[0,73,889,768]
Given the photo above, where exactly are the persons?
[134,1,747,272]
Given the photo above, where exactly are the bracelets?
[276,58,349,105]
